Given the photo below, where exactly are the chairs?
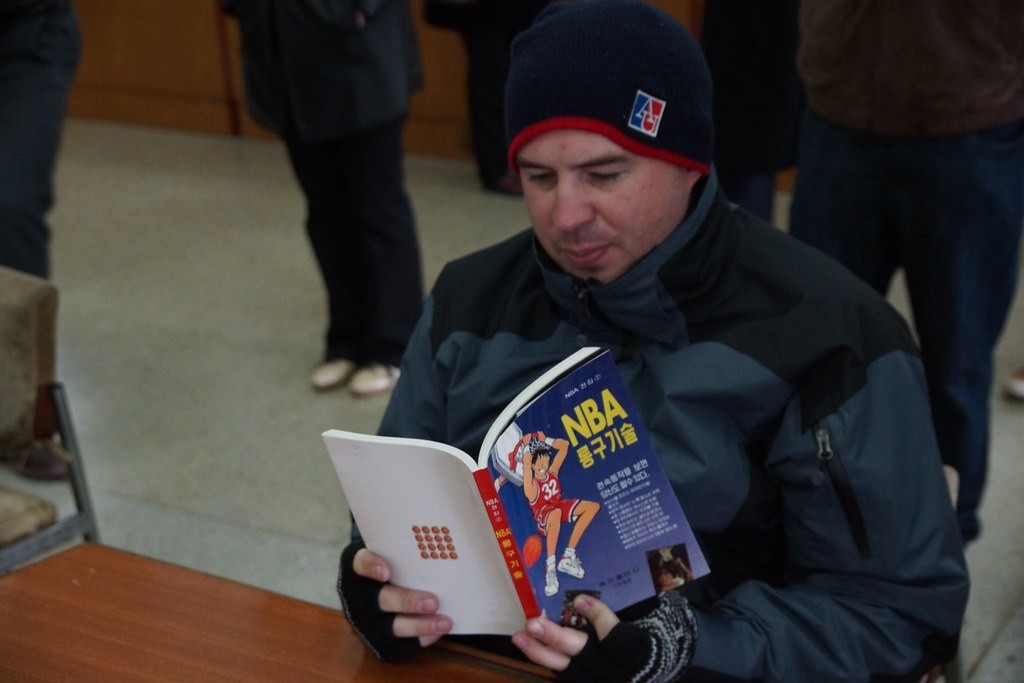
[0,267,97,576]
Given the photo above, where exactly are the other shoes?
[1006,368,1023,400]
[5,442,70,477]
[481,172,525,197]
[311,351,356,390]
[348,358,402,394]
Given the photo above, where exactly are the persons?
[786,0,1024,545]
[424,0,552,195]
[0,0,84,480]
[336,0,970,683]
[695,0,805,223]
[217,0,426,396]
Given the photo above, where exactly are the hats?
[503,2,715,174]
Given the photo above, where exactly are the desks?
[0,543,559,683]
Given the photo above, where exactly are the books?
[322,347,710,634]
